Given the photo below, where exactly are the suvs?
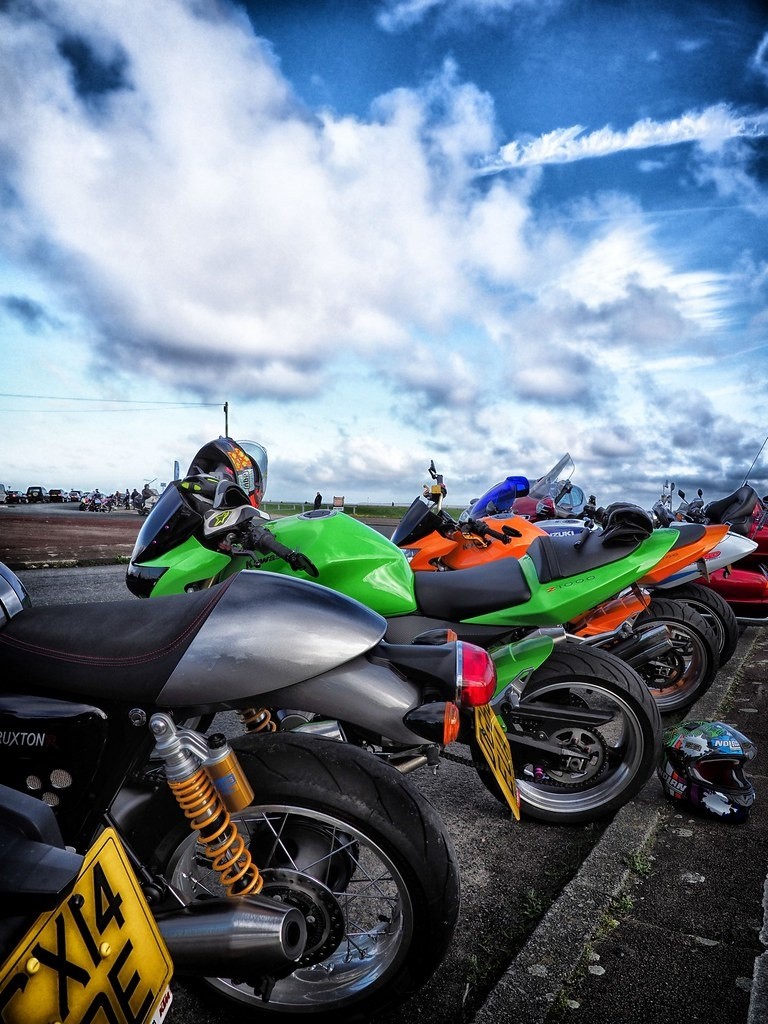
[0,484,11,505]
[26,486,50,503]
[48,487,70,503]
[71,490,83,498]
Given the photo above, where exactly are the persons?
[124,489,130,510]
[313,492,322,509]
[115,491,121,502]
[141,484,156,508]
[93,489,101,498]
[131,489,139,499]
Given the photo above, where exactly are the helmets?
[656,719,759,823]
[186,435,268,508]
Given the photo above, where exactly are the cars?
[6,490,29,504]
[70,492,81,502]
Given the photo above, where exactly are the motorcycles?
[0,451,767,1024]
